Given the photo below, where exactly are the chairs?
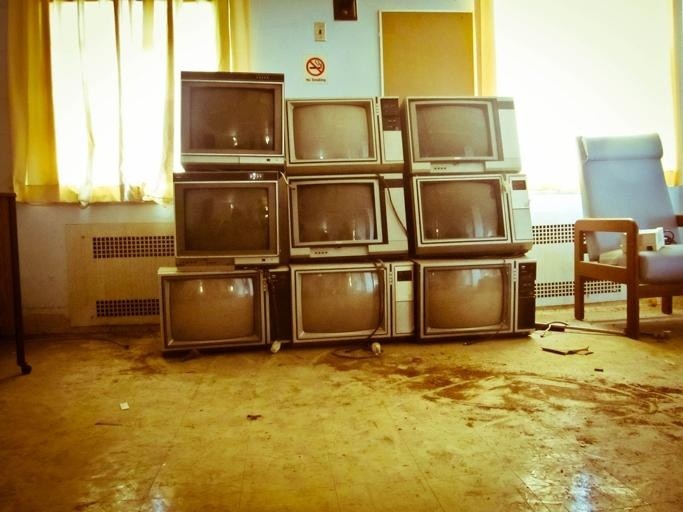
[574,133,683,336]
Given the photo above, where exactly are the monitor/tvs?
[285,96,405,175]
[287,173,409,263]
[156,264,292,353]
[173,171,281,272]
[411,255,536,340]
[289,261,414,345]
[181,71,286,171]
[408,174,533,258]
[399,95,520,177]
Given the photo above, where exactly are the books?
[543,343,589,355]
